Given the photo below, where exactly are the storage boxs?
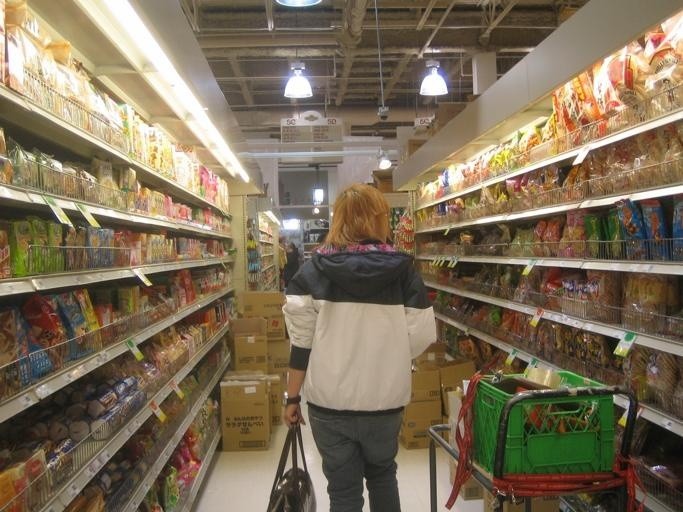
[482,487,560,512]
[219,292,291,450]
[399,343,476,451]
[446,389,483,499]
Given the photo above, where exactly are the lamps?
[418,43,449,96]
[282,47,313,98]
[271,0,323,11]
[376,146,391,170]
[310,166,324,206]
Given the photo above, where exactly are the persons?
[278,236,287,292]
[282,242,300,287]
[281,182,439,511]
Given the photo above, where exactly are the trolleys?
[429,369,641,512]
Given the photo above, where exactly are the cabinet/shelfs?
[0,2,237,512]
[299,218,329,265]
[258,197,283,293]
[391,80,683,512]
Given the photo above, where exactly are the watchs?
[282,393,302,407]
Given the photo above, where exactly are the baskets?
[473,371,614,475]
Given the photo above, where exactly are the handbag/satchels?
[267,423,316,512]
